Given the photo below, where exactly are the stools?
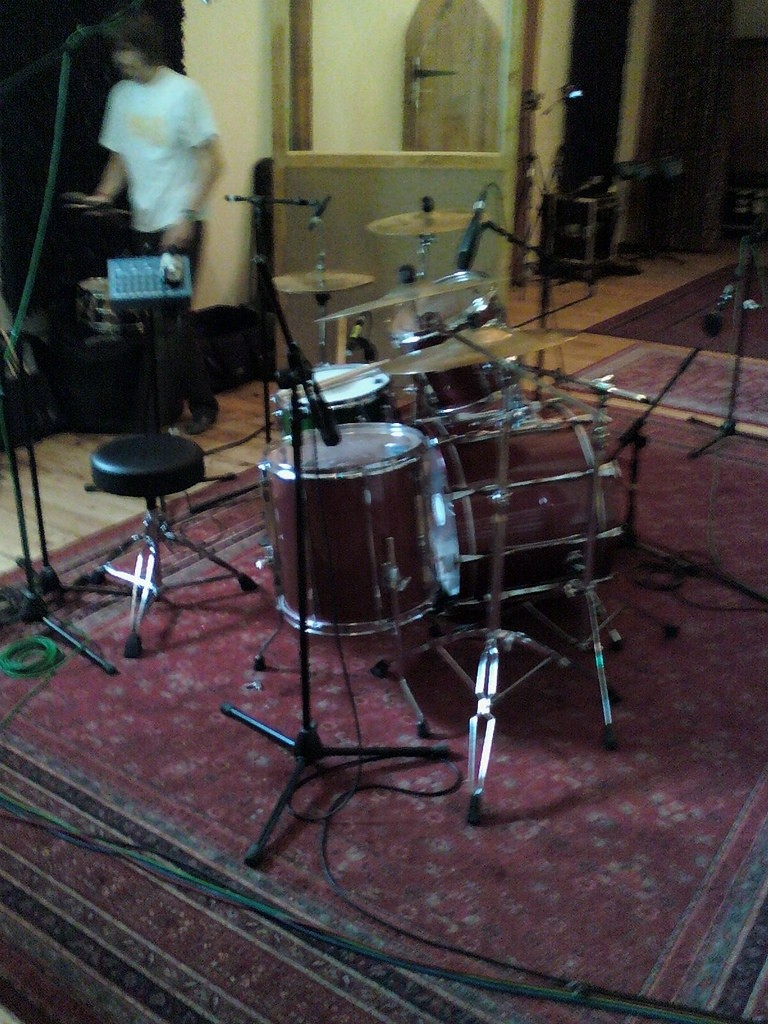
[66,438,255,660]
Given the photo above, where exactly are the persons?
[60,5,226,439]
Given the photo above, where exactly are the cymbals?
[379,324,586,377]
[366,206,489,238]
[313,274,543,323]
[270,270,374,295]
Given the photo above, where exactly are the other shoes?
[189,416,214,434]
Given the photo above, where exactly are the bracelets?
[180,209,198,223]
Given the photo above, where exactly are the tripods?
[220,192,768,866]
[0,15,143,674]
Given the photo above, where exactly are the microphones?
[308,194,330,229]
[346,311,368,356]
[458,189,487,272]
[701,283,739,338]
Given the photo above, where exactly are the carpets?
[0,387,768,1024]
[556,344,768,427]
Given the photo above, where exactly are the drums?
[398,381,525,448]
[266,419,444,640]
[78,275,149,334]
[416,394,613,604]
[274,362,391,436]
